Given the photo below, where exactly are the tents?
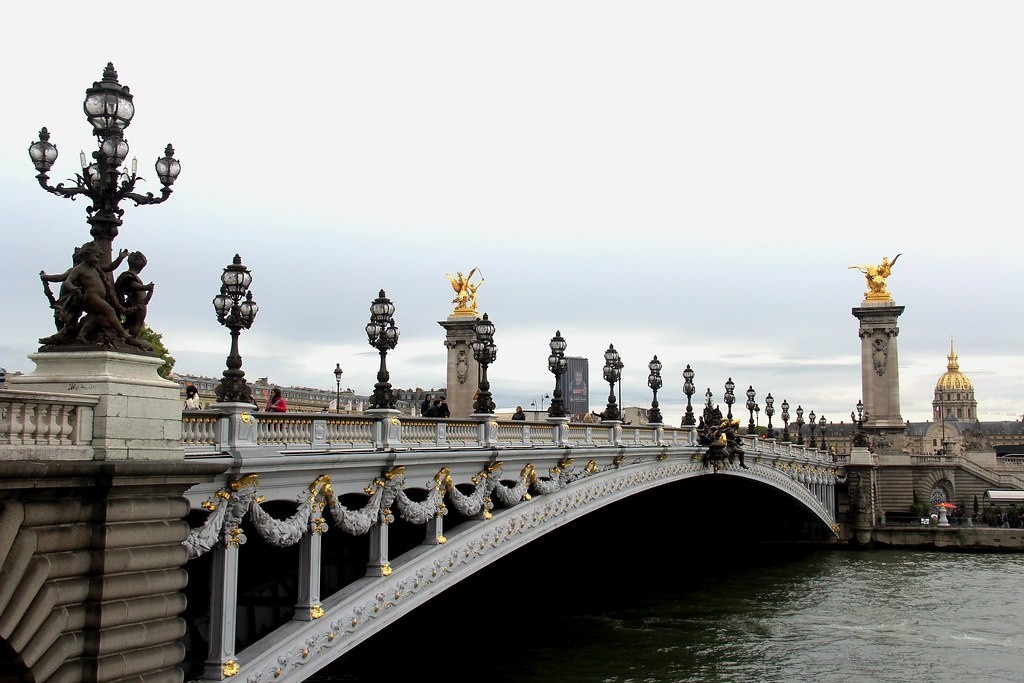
[983,490,1024,508]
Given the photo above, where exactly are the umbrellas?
[935,502,956,509]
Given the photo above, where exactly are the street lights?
[723,376,736,424]
[765,392,775,438]
[796,405,805,445]
[467,312,497,414]
[541,393,550,410]
[547,329,567,416]
[745,385,756,434]
[808,410,828,450]
[333,363,343,414]
[211,252,260,406]
[531,399,537,410]
[851,399,871,447]
[599,342,624,421]
[705,388,713,407]
[781,399,791,441]
[681,364,697,425]
[27,61,182,354]
[365,286,400,409]
[755,403,760,434]
[648,354,663,424]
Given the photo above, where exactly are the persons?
[622,417,632,425]
[592,411,604,424]
[41,241,154,349]
[453,271,482,310]
[916,449,946,459]
[184,380,200,410]
[265,388,287,432]
[923,509,1024,529]
[511,406,526,420]
[421,394,450,417]
[703,418,749,469]
[878,253,904,278]
[697,416,704,429]
